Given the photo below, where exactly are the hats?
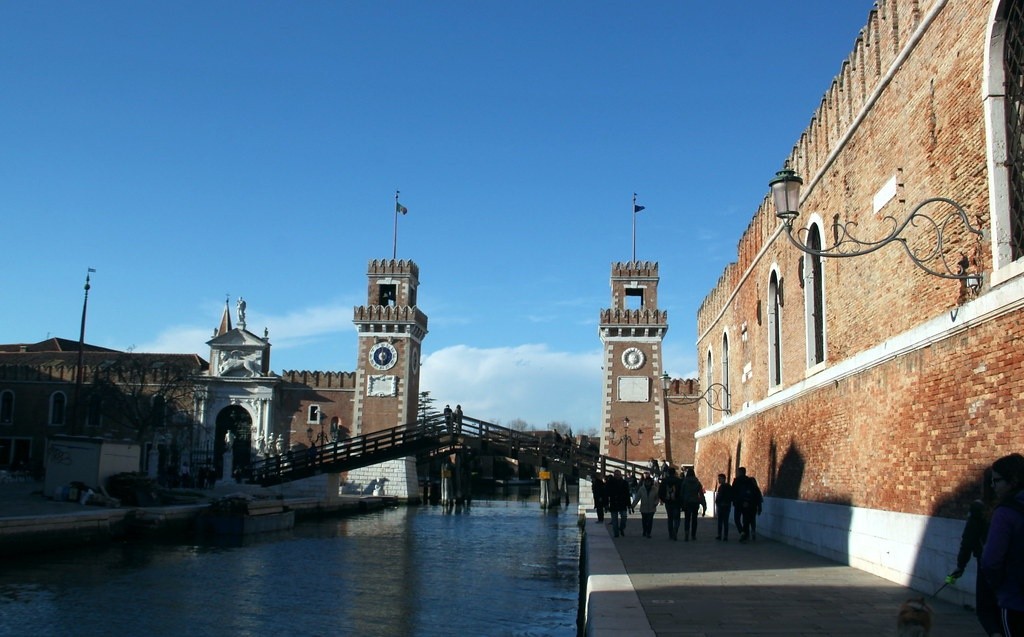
[993,453,1023,483]
[591,474,596,478]
[738,467,746,475]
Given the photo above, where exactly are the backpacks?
[658,478,676,505]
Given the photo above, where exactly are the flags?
[633,204,645,213]
[88,266,97,272]
[396,201,408,217]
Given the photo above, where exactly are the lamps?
[769,159,986,296]
[659,371,732,414]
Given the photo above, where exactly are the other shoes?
[691,534,696,541]
[684,532,690,541]
[647,533,651,538]
[620,528,625,536]
[668,527,673,538]
[673,529,678,540]
[642,532,646,536]
[614,535,619,537]
[739,532,745,541]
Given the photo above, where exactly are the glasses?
[993,477,1004,484]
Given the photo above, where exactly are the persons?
[732,466,765,544]
[224,428,237,452]
[453,405,462,433]
[680,468,706,541]
[562,433,572,459]
[257,430,268,460]
[553,430,562,452]
[714,472,733,542]
[237,296,246,321]
[591,470,634,538]
[632,459,686,540]
[444,404,454,434]
[266,432,277,456]
[276,435,285,456]
[180,461,190,485]
[587,441,599,467]
[979,453,1024,637]
[197,462,218,492]
[951,464,1005,637]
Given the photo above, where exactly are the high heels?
[716,535,721,542]
[723,537,729,544]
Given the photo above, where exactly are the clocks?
[369,342,399,371]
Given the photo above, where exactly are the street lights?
[609,416,644,481]
